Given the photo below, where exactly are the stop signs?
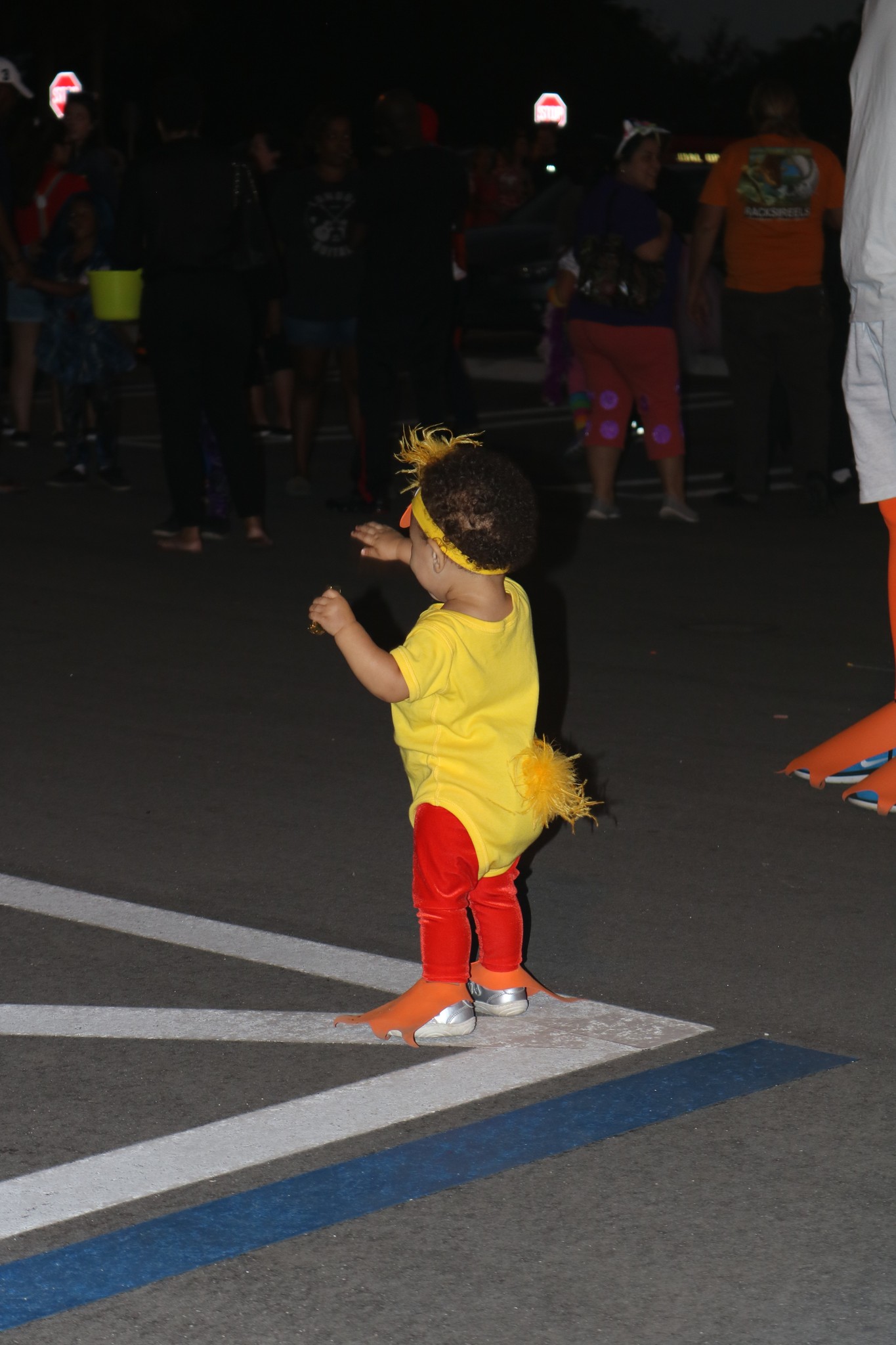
[534,93,567,130]
[49,71,82,118]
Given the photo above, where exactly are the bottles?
[549,249,579,308]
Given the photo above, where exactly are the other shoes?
[275,468,307,494]
[587,504,620,519]
[806,491,832,511]
[327,464,389,513]
[148,522,205,555]
[331,977,479,1048]
[465,958,578,1015]
[659,500,699,524]
[225,511,265,543]
[841,758,896,817]
[258,420,296,450]
[1,423,131,492]
[200,513,232,543]
[714,492,764,513]
[774,700,895,789]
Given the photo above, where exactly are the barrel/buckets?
[86,268,144,320]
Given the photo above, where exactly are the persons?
[779,0,896,817]
[306,422,604,1049]
[0,84,580,555]
[682,78,849,492]
[567,118,705,525]
